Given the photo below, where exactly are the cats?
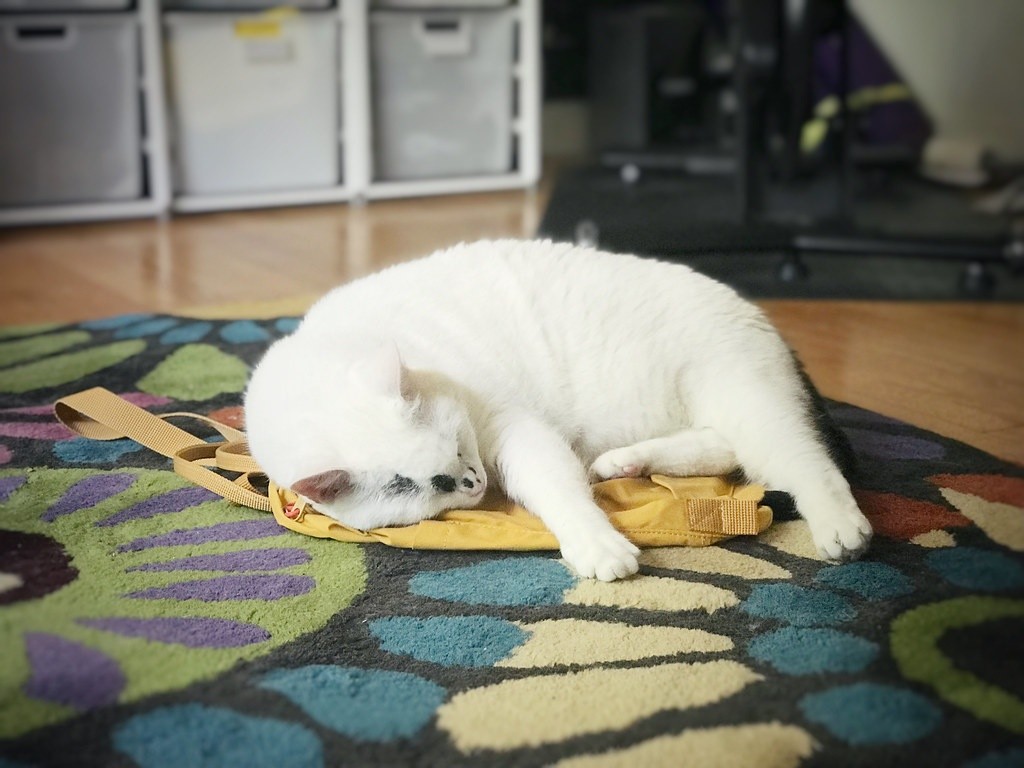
[240,239,874,585]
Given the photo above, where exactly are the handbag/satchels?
[52,386,774,551]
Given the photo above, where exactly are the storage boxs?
[167,7,345,197]
[370,8,515,179]
[0,18,143,207]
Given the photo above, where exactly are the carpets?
[0,297,1024,768]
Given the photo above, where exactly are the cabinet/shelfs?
[0,0,544,228]
